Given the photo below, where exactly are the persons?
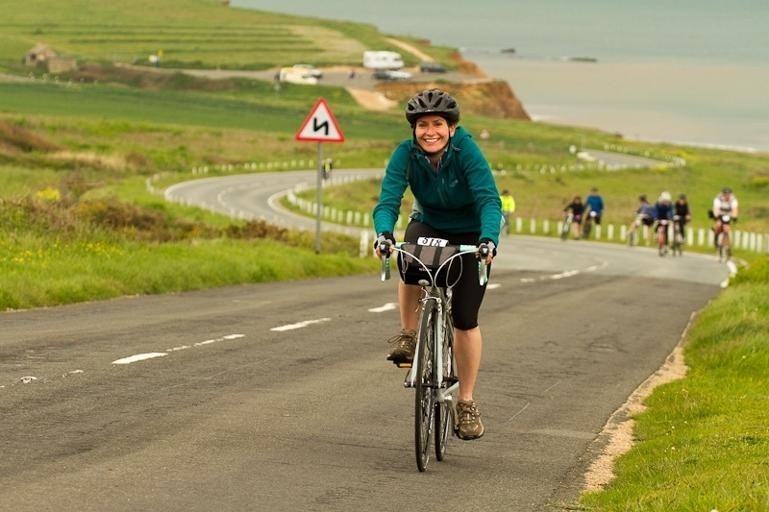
[368,89,506,444]
[670,193,692,250]
[559,194,584,241]
[584,185,604,225]
[627,192,654,241]
[711,186,739,259]
[649,192,674,254]
[497,188,516,235]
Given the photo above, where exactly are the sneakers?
[456,398,485,441]
[387,327,419,361]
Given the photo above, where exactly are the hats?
[721,187,731,194]
[658,192,689,203]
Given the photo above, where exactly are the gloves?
[477,239,497,265]
[373,233,395,256]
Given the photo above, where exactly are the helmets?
[403,88,460,124]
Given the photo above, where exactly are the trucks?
[362,50,404,71]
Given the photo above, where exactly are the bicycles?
[673,216,685,258]
[582,210,598,239]
[558,211,572,238]
[374,238,492,473]
[654,220,672,258]
[711,216,740,264]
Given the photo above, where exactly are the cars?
[370,69,410,81]
[292,61,323,79]
[415,62,448,73]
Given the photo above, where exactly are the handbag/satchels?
[399,243,462,287]
